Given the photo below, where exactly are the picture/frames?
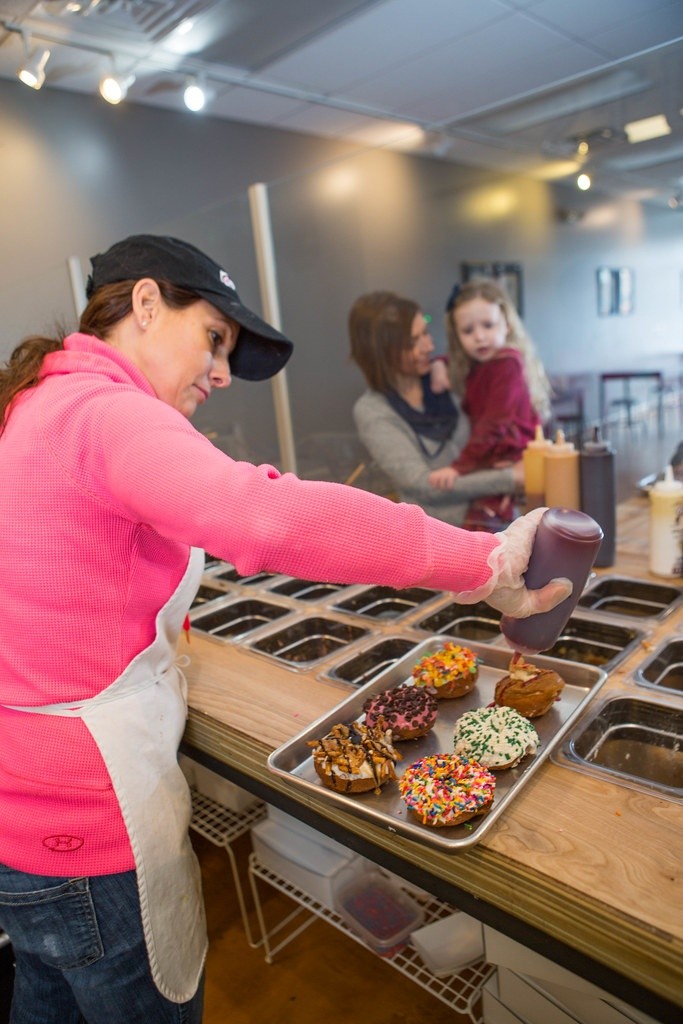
[461,261,524,321]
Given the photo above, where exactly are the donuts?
[305,721,404,794]
[398,752,496,826]
[454,705,540,770]
[412,641,484,698]
[361,683,438,741]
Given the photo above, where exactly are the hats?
[83,233,295,388]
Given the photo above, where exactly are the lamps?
[182,72,219,111]
[17,32,50,90]
[99,71,135,105]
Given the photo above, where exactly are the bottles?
[542,430,580,512]
[499,507,605,665]
[649,465,683,579]
[523,426,553,514]
[578,428,615,568]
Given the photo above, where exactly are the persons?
[348,290,523,534]
[425,274,556,532]
[0,232,576,1024]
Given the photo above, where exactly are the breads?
[494,656,564,717]
